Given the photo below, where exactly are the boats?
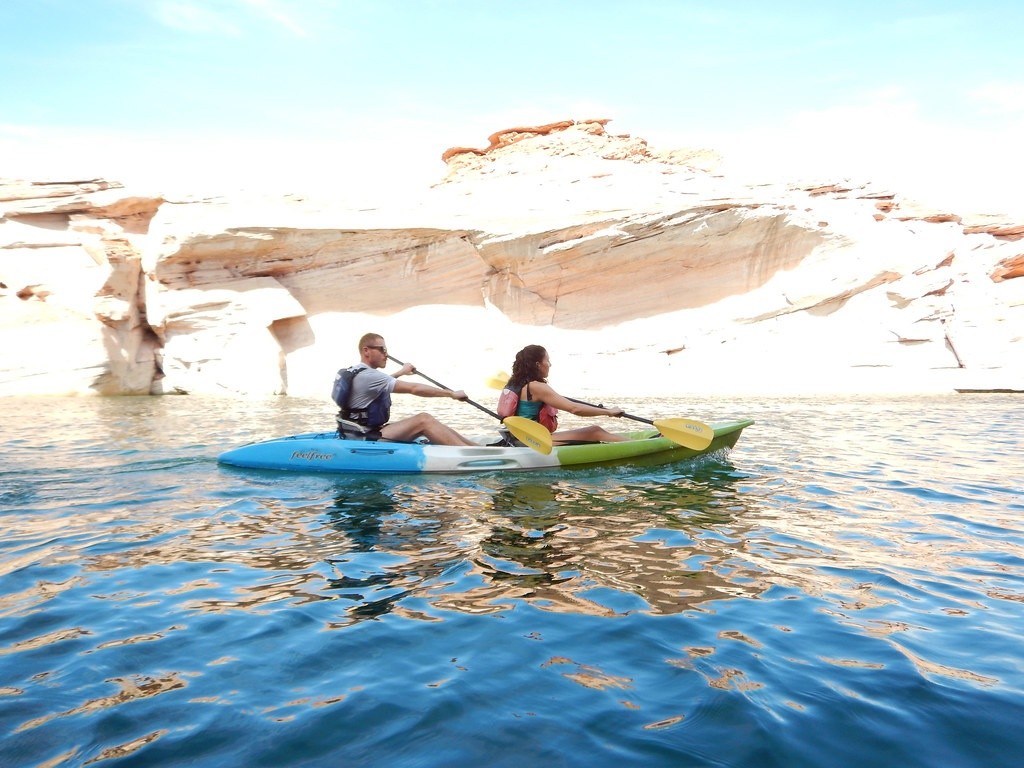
[217,418,756,473]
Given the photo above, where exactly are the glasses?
[365,346,387,354]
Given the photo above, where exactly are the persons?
[497,345,664,445]
[331,333,506,447]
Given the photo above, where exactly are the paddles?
[387,354,554,456]
[488,371,715,452]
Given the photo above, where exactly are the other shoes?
[486,438,506,446]
[649,433,662,438]
